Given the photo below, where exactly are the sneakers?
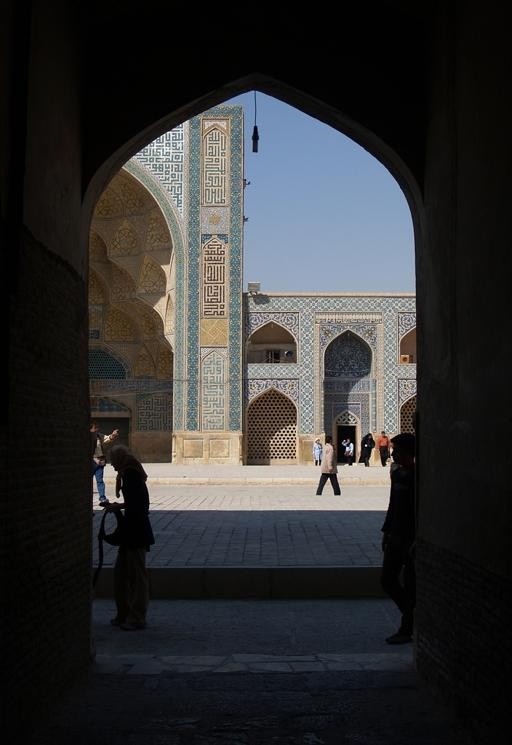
[385,633,413,645]
[110,616,147,631]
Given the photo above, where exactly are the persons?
[376,431,391,467]
[312,438,322,466]
[315,436,341,496]
[107,443,151,519]
[341,438,355,467]
[90,420,120,506]
[360,433,375,467]
[379,434,414,646]
